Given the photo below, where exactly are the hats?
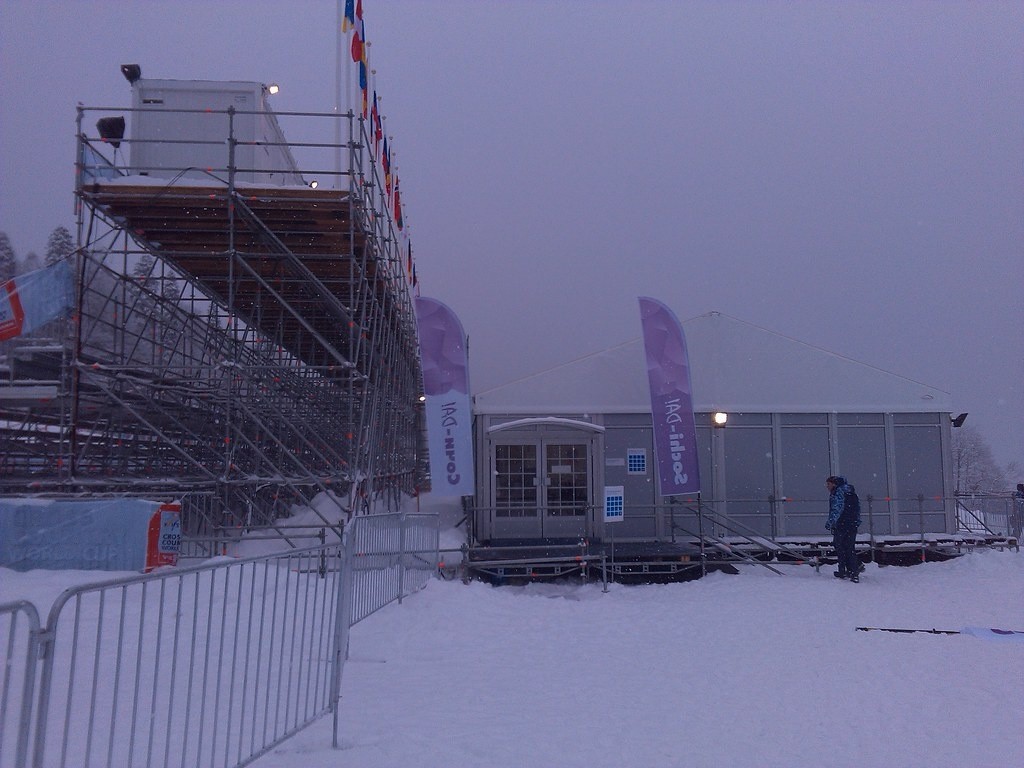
[826,476,837,484]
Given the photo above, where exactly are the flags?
[341,0,418,290]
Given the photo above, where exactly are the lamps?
[309,177,312,181]
[120,63,141,84]
[713,413,728,428]
[266,83,279,96]
[950,413,968,428]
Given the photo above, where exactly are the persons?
[825,475,866,583]
[1007,484,1024,547]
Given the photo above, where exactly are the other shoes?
[834,571,846,579]
[850,576,858,582]
